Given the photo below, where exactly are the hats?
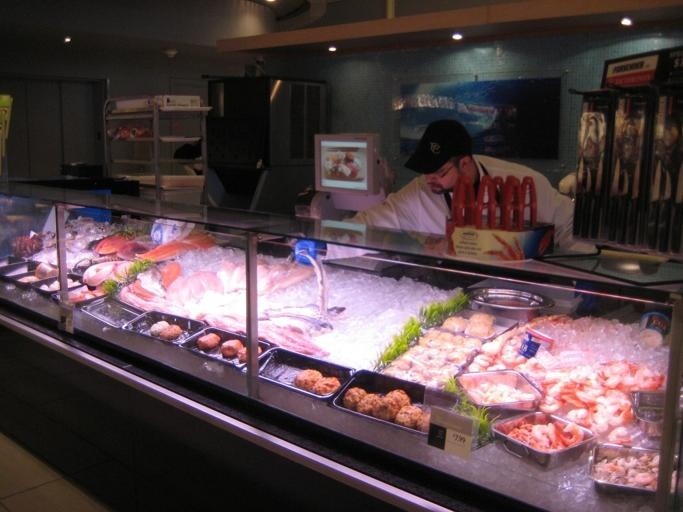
[404,120,472,174]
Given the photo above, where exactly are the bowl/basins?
[629,390,665,438]
[463,286,556,324]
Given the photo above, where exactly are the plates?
[159,106,212,112]
[82,261,137,288]
[160,136,201,142]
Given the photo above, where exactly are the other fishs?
[248,300,348,359]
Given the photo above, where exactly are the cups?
[517,326,554,359]
[639,312,671,342]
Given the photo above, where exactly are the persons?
[340,118,596,253]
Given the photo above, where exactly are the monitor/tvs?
[313,134,376,194]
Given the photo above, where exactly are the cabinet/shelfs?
[102,95,213,205]
